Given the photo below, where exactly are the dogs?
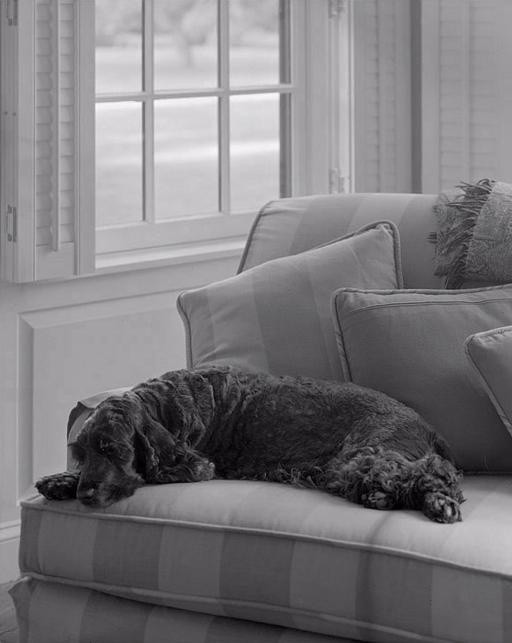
[33,363,467,524]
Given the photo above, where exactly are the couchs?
[7,190,512,642]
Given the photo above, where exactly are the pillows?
[333,284,512,476]
[177,219,405,386]
[463,325,512,441]
